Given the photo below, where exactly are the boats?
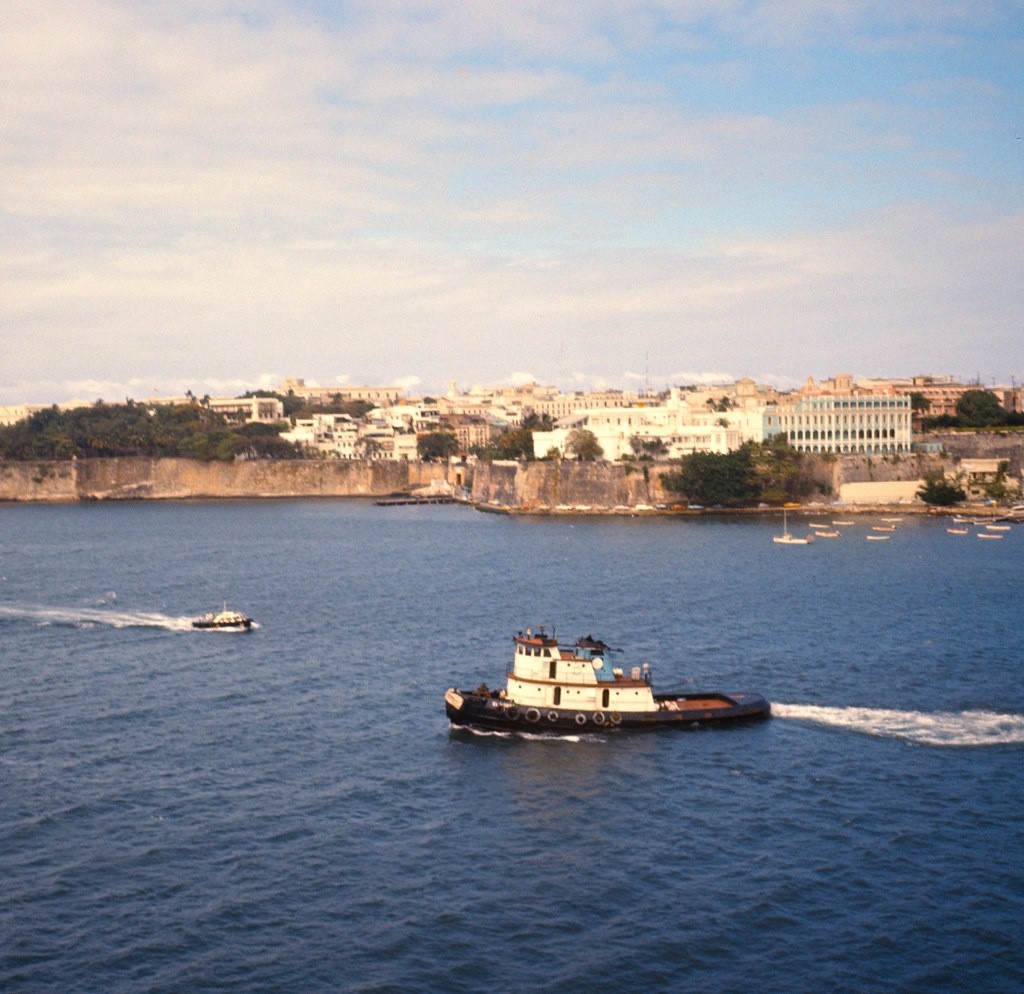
[831,521,855,526]
[977,533,1004,540]
[987,525,1012,531]
[946,528,968,534]
[814,530,842,538]
[953,514,1006,525]
[881,518,903,523]
[443,624,773,736]
[772,507,812,545]
[192,600,254,630]
[808,523,830,529]
[866,535,890,541]
[872,525,898,532]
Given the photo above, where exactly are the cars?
[854,499,924,506]
[831,501,848,506]
[655,504,670,510]
[475,495,551,511]
[576,505,593,511]
[597,505,611,511]
[670,504,687,511]
[807,501,824,506]
[616,505,631,511]
[556,504,572,509]
[985,500,999,506]
[1010,505,1024,514]
[783,501,801,508]
[688,504,705,510]
[636,505,653,512]
[712,503,725,509]
[757,502,770,508]
